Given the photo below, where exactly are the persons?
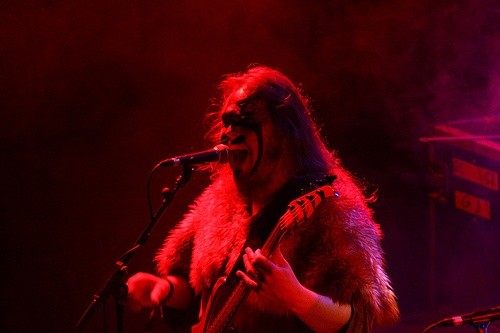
[118,59,401,333]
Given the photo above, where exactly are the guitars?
[191,184,335,333]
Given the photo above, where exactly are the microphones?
[159,144,231,164]
[439,306,500,326]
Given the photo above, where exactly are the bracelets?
[150,275,174,319]
